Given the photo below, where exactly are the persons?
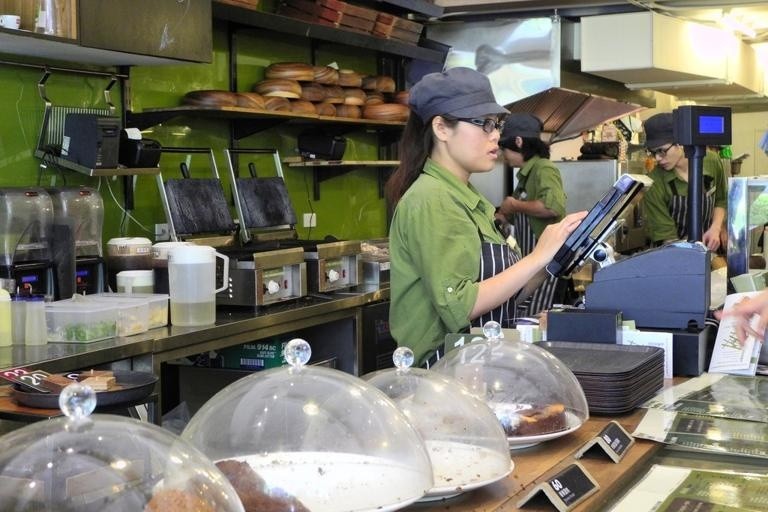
[384,67,590,374]
[712,292,767,341]
[491,114,572,320]
[639,110,730,261]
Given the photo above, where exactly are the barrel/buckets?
[152,241,197,324]
[116,270,156,294]
[105,237,153,293]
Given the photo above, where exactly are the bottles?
[0,288,48,347]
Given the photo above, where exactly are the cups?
[113,267,154,295]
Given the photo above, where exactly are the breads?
[182,62,412,122]
[144,487,217,512]
[498,402,578,430]
[40,369,124,394]
[216,461,308,512]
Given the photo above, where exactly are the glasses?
[457,118,506,134]
[646,143,675,159]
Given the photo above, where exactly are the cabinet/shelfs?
[121,0,447,208]
[0,0,210,73]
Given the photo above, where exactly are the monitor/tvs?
[545,173,655,279]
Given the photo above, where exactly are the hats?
[498,111,543,144]
[406,67,511,127]
[644,113,674,149]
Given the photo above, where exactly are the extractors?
[427,15,656,150]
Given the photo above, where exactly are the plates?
[211,450,434,512]
[420,435,513,501]
[485,402,581,452]
